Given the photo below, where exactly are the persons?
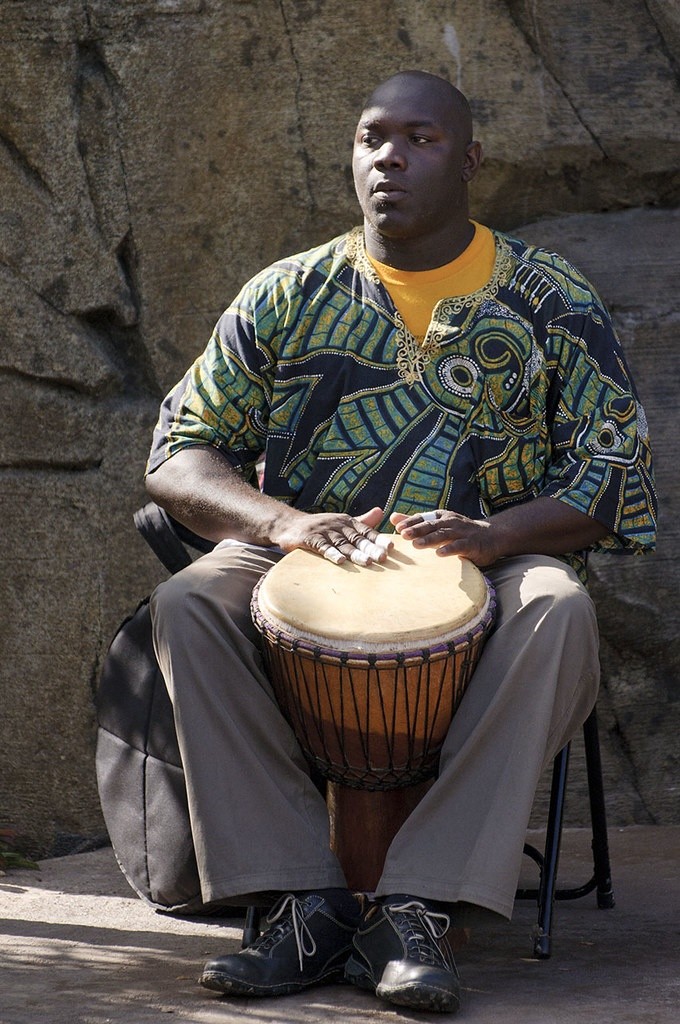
[140,69,662,1011]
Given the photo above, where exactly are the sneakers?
[342,899,463,1012]
[198,889,371,996]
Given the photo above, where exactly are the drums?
[248,530,501,890]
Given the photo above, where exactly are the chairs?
[241,657,616,964]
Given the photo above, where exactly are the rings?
[419,511,437,521]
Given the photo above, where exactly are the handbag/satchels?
[95,500,221,913]
